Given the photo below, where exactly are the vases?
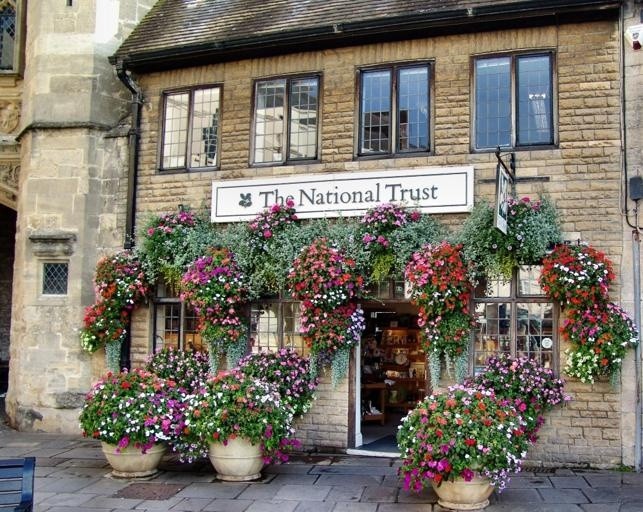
[430,479,496,510]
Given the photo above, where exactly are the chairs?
[0,455,37,511]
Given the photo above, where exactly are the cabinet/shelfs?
[361,328,427,427]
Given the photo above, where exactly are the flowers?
[68,189,641,498]
